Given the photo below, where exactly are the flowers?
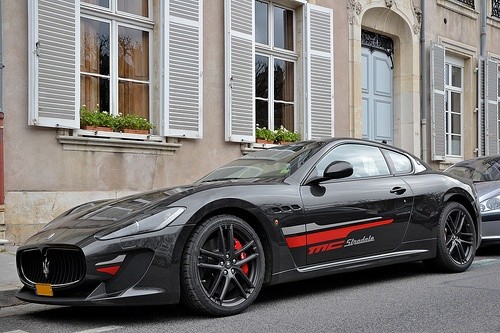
[256,124,301,141]
[116,111,156,133]
[79,104,115,128]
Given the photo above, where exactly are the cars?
[442,156,500,247]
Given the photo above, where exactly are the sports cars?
[16,138,486,317]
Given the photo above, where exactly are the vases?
[84,126,112,132]
[257,138,274,144]
[280,140,295,145]
[123,128,149,135]
